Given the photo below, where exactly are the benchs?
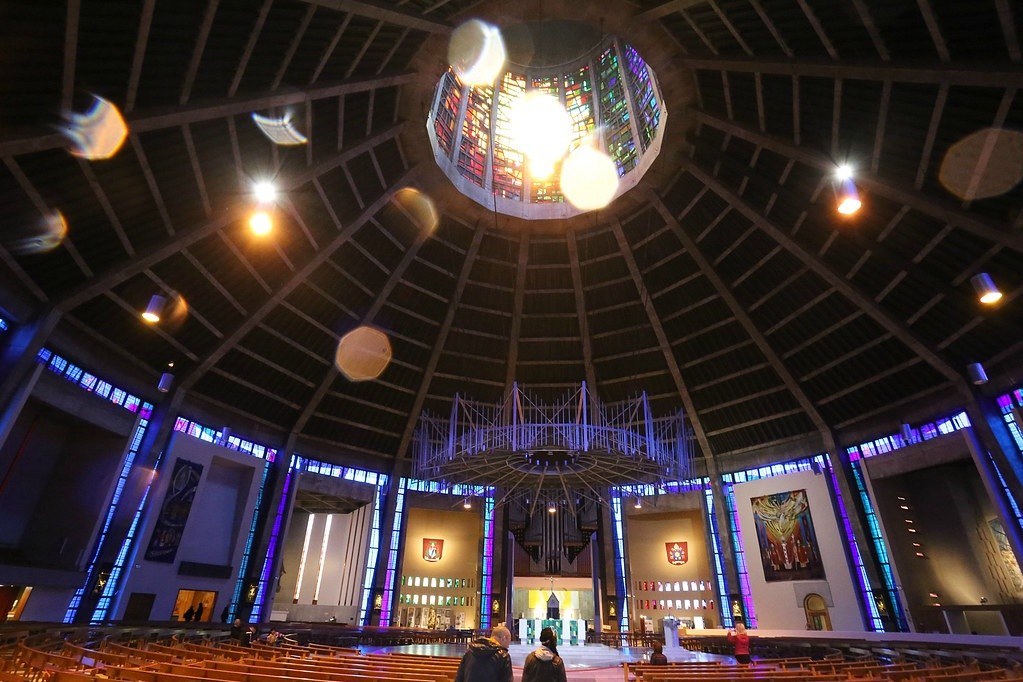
[624,648,1023,682]
[0,634,463,682]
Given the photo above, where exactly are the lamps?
[464,498,641,513]
[142,291,167,322]
[970,272,1003,304]
[966,362,988,385]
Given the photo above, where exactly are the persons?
[727,623,751,665]
[220,603,231,631]
[650,641,667,664]
[184,606,195,621]
[231,618,286,648]
[455,625,513,682]
[521,627,567,682]
[194,602,203,621]
